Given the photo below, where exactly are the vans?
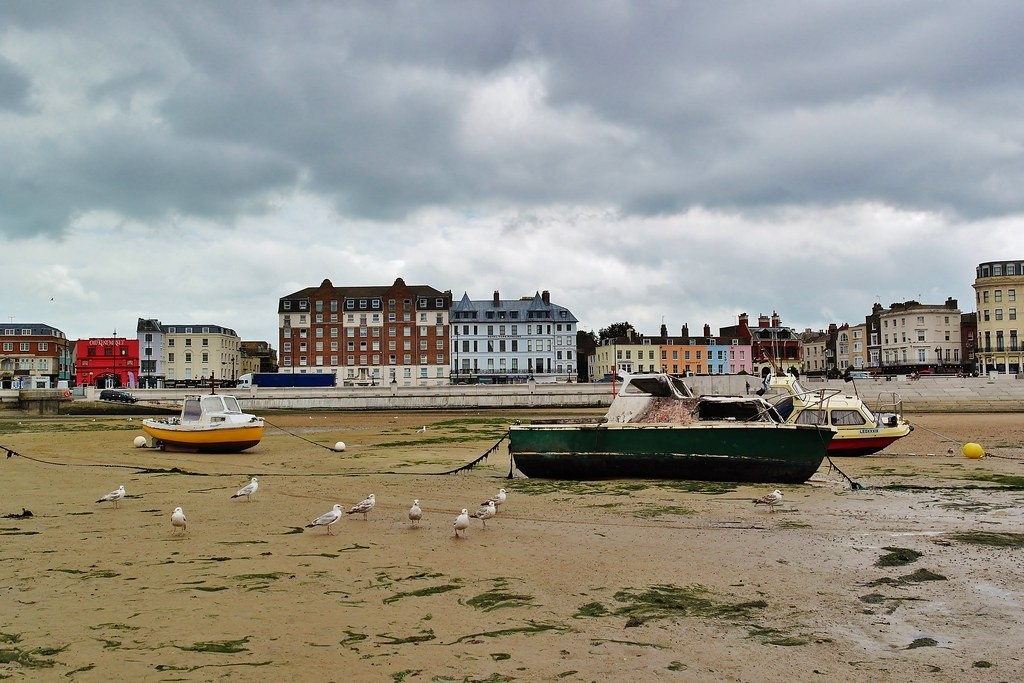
[919,370,934,376]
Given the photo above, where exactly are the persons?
[746,381,751,395]
[910,370,920,381]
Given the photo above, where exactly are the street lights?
[456,332,461,385]
[64,337,67,380]
[113,329,117,388]
[292,329,297,387]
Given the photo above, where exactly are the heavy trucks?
[236,371,336,388]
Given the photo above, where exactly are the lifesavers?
[64,391,70,397]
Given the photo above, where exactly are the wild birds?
[127,418,132,422]
[948,447,954,456]
[752,489,784,514]
[469,501,496,528]
[489,489,509,513]
[454,508,470,538]
[230,478,259,503]
[344,494,375,521]
[409,499,423,528]
[95,485,125,509]
[171,507,187,537]
[416,426,426,434]
[305,504,345,536]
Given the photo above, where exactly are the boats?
[141,392,263,452]
[509,371,836,482]
[764,376,915,456]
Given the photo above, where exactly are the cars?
[457,382,466,385]
[99,390,137,404]
